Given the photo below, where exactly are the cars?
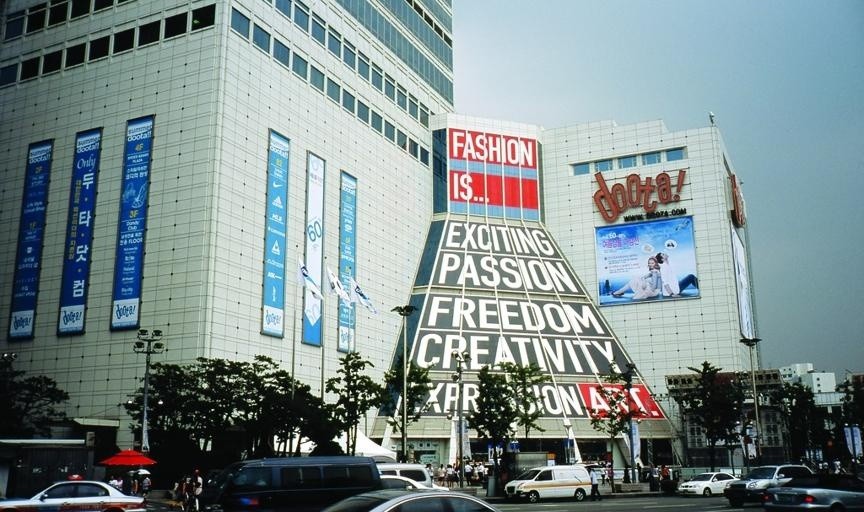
[0,480,147,512]
[380,474,431,490]
[677,472,741,497]
[321,488,503,512]
[764,475,864,511]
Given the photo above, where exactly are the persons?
[656,252,698,298]
[801,453,864,476]
[587,454,673,501]
[425,460,489,489]
[611,257,660,300]
[108,467,221,512]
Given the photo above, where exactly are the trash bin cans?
[649,473,660,492]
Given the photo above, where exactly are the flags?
[349,277,377,315]
[325,263,353,309]
[297,259,324,301]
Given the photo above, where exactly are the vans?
[503,463,601,502]
[375,462,433,489]
[198,457,383,512]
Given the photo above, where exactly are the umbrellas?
[98,448,157,466]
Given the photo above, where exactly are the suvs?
[724,464,815,507]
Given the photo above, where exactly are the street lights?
[391,305,418,457]
[834,384,858,464]
[511,428,518,453]
[739,337,768,457]
[447,350,471,488]
[563,423,573,464]
[133,327,165,452]
[625,362,637,485]
